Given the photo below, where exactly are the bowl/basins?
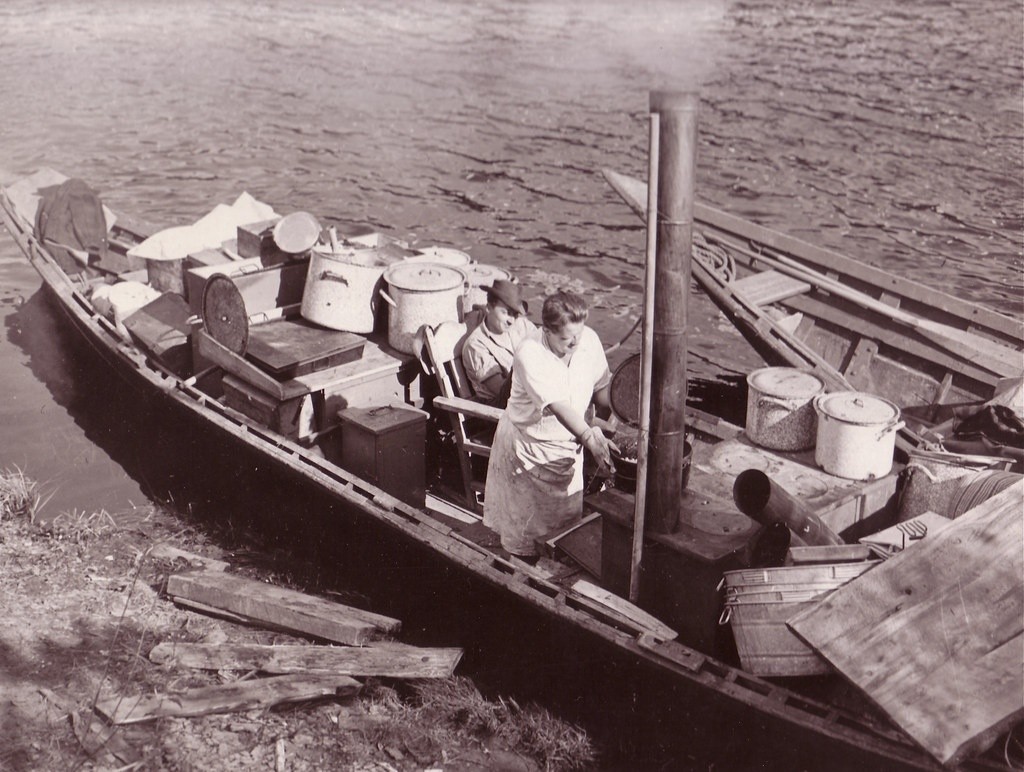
[608,433,693,493]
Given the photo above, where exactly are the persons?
[462,279,537,408]
[482,290,621,567]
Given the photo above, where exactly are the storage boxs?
[236,217,280,258]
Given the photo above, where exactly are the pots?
[299,232,520,354]
[811,391,907,482]
[746,366,826,452]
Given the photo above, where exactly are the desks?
[583,432,905,667]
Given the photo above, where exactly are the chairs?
[424,310,502,510]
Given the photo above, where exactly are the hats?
[479,279,525,314]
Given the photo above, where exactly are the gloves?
[581,425,621,488]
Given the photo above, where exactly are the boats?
[1,92,1024,772]
[600,164,1024,470]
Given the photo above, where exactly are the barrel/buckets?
[717,561,878,676]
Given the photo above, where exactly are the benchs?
[736,269,818,308]
